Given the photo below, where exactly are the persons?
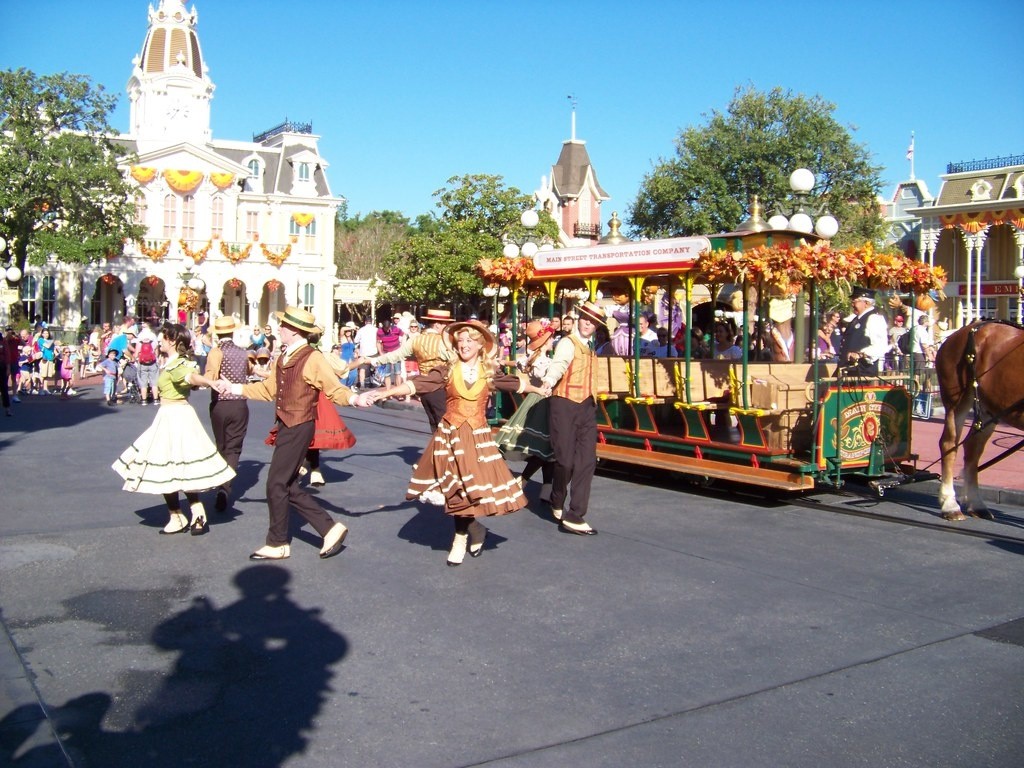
[0,315,122,416]
[805,285,888,386]
[111,302,606,567]
[884,315,936,415]
[595,282,793,365]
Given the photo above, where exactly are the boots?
[190,501,207,535]
[467,519,489,556]
[159,509,188,534]
[539,483,553,508]
[516,475,527,489]
[447,533,468,566]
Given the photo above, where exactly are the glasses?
[411,326,418,328]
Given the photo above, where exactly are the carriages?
[483,228,1024,523]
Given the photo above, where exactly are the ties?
[588,341,593,351]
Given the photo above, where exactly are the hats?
[391,313,402,320]
[274,307,325,333]
[526,321,559,350]
[106,348,119,358]
[895,315,904,322]
[123,327,138,338]
[574,301,608,327]
[442,320,493,354]
[207,316,240,333]
[420,309,456,322]
[849,286,876,299]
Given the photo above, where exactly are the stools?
[912,391,941,420]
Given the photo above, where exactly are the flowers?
[690,236,950,318]
[476,255,534,287]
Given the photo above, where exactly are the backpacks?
[140,340,156,365]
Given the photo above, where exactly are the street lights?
[481,282,511,323]
[764,168,838,365]
[498,207,556,325]
[173,262,205,329]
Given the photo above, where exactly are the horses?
[936,319,1024,521]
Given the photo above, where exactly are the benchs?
[594,354,853,453]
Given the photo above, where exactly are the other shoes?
[298,457,326,486]
[562,519,597,536]
[215,490,228,512]
[319,521,348,557]
[5,385,72,416]
[105,384,161,406]
[550,508,562,522]
[250,544,291,559]
